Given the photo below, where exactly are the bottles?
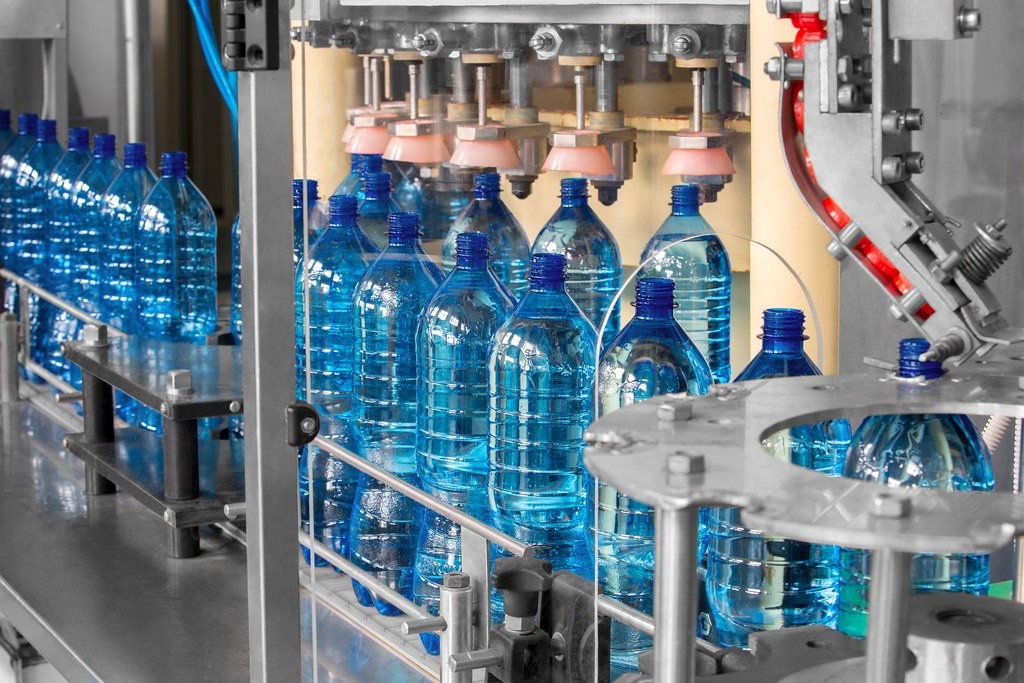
[133,152,217,438]
[0,109,17,158]
[232,179,472,617]
[835,339,994,640]
[418,172,531,655]
[0,113,177,433]
[705,306,851,651]
[486,177,622,622]
[582,185,731,673]
